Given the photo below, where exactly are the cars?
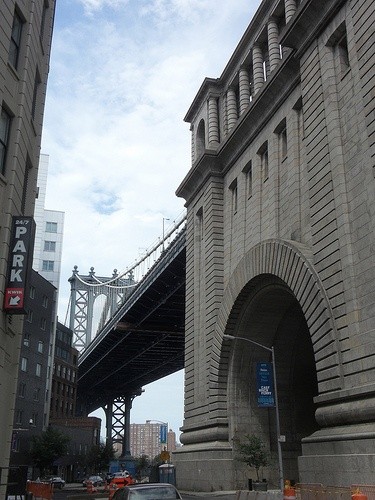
[113,483,182,500]
[83,476,105,487]
[39,476,66,489]
[107,471,130,484]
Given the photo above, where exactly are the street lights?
[221,332,284,498]
[162,217,170,250]
[146,418,171,483]
